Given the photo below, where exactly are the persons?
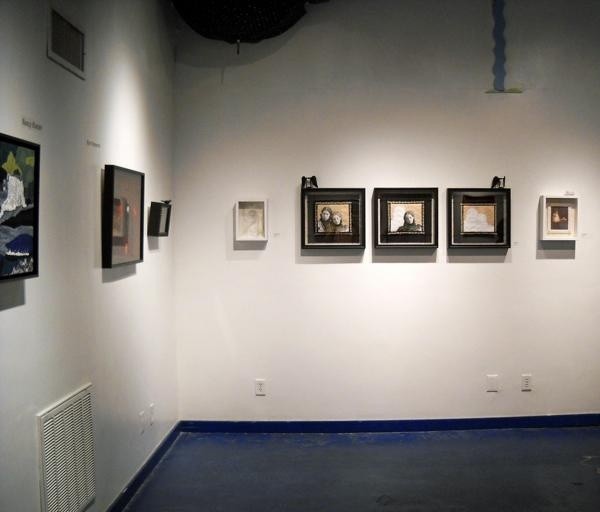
[397,211,424,233]
[317,206,334,233]
[330,212,349,233]
[464,207,479,232]
[475,214,495,233]
[242,209,259,241]
[552,209,561,229]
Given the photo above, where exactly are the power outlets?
[519,374,533,392]
[254,377,268,396]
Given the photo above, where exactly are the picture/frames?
[100,163,146,269]
[299,186,367,251]
[232,197,271,243]
[1,132,42,284]
[46,4,90,82]
[147,201,174,238]
[538,190,582,244]
[444,186,513,250]
[372,185,441,251]
